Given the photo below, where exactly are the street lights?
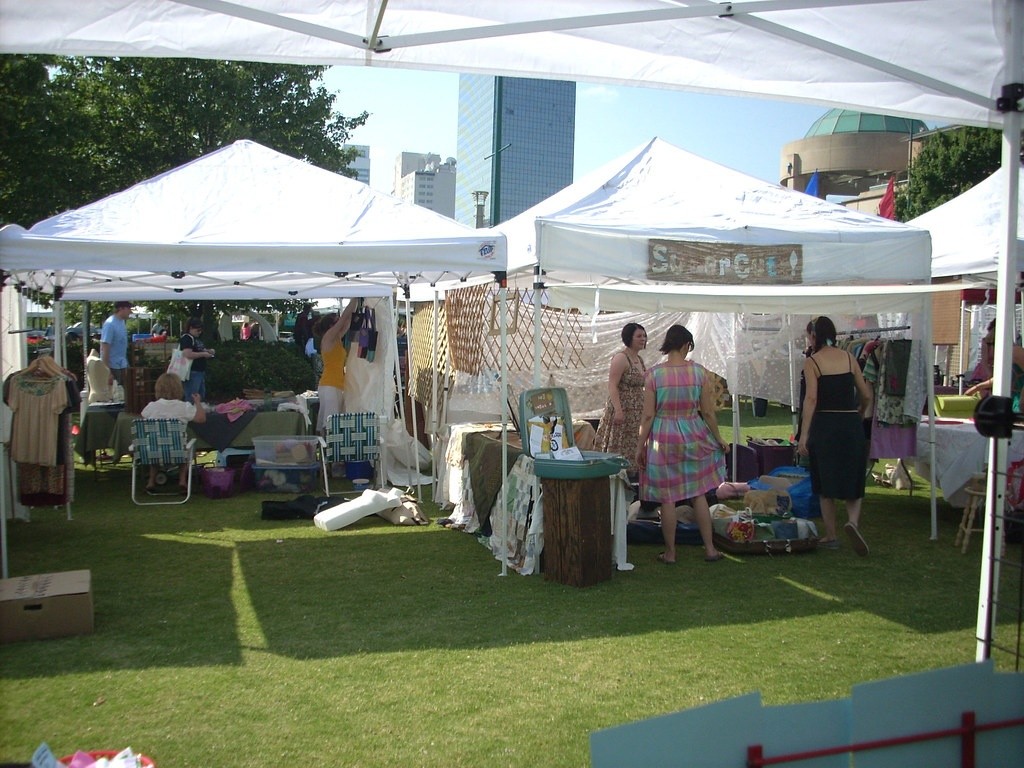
[471,191,491,228]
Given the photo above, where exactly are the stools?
[955,489,1008,556]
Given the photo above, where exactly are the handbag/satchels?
[167,334,194,382]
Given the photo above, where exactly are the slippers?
[179,489,187,494]
[656,551,676,564]
[141,485,155,493]
[704,552,722,561]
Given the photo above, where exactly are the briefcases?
[520,387,631,479]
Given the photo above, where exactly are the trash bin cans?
[746,438,797,476]
[518,387,629,587]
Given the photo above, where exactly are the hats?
[115,301,136,308]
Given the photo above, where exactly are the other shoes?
[843,522,869,556]
[816,537,838,550]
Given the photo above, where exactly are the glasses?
[197,328,202,333]
[811,315,821,336]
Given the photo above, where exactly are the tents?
[907,163,1024,279]
[0,138,509,576]
[396,137,935,541]
[958,289,1020,396]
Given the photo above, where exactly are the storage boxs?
[746,438,796,475]
[253,463,318,492]
[253,435,318,466]
[0,570,94,645]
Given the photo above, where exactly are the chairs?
[318,412,386,499]
[130,417,198,506]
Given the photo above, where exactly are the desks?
[917,415,1024,480]
[116,408,303,445]
[80,407,138,478]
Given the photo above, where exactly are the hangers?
[11,356,77,384]
[837,326,911,343]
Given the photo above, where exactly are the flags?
[805,172,818,198]
[878,179,894,219]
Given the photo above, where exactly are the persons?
[592,323,648,477]
[242,323,258,339]
[794,316,868,556]
[964,318,1024,412]
[45,323,53,337]
[635,324,730,560]
[100,301,132,387]
[180,316,216,403]
[294,305,311,343]
[151,320,168,336]
[141,373,207,493]
[316,297,364,433]
